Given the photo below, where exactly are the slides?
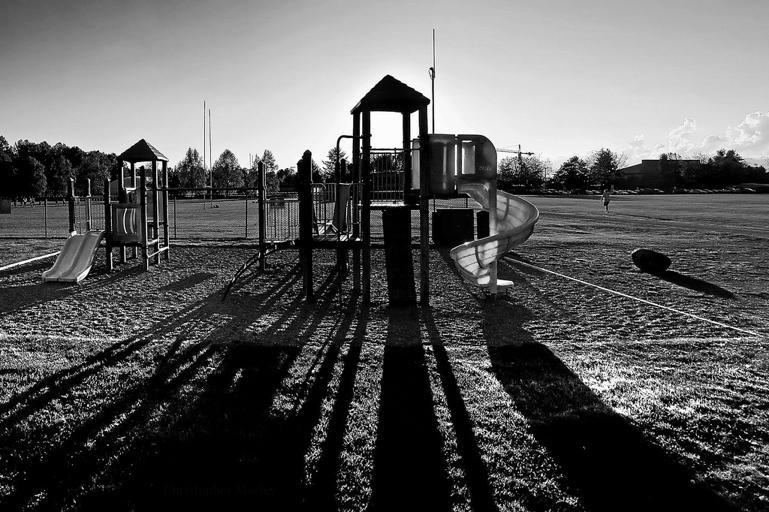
[41,227,104,283]
[449,182,540,289]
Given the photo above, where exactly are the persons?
[601,187,613,212]
